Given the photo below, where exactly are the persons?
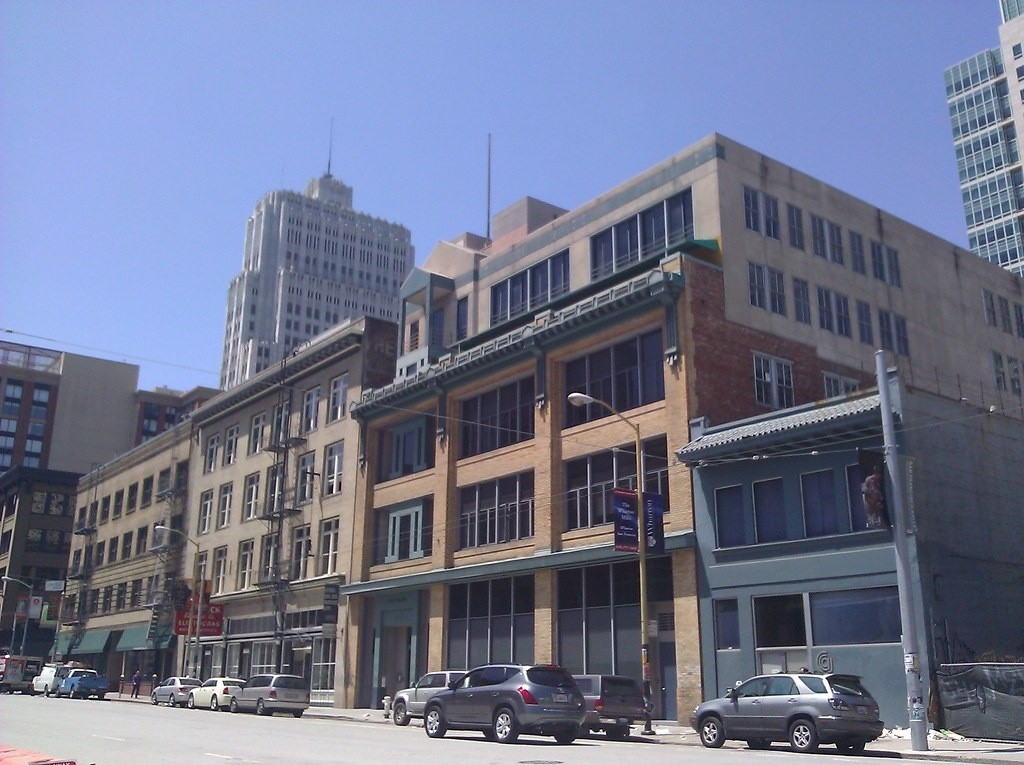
[131,671,140,698]
[196,579,209,604]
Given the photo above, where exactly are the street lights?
[2,576,33,656]
[567,393,656,735]
[155,526,199,677]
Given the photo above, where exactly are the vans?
[30,663,81,697]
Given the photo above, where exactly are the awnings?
[116,627,176,651]
[48,630,111,656]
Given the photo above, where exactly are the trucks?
[0,655,45,695]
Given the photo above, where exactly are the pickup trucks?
[56,669,111,699]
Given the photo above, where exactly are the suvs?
[229,674,311,718]
[689,667,884,753]
[554,675,640,738]
[392,669,470,726]
[424,663,586,744]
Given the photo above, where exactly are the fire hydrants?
[381,696,392,719]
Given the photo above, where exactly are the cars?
[151,677,203,707]
[187,678,246,711]
